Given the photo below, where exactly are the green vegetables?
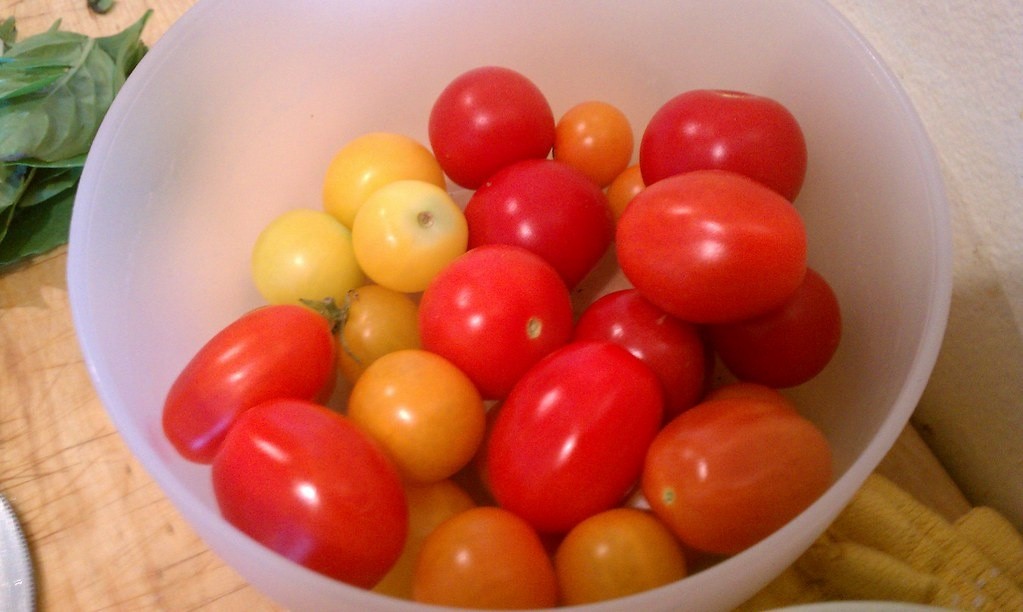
[0,0,155,266]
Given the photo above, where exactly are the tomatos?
[162,65,843,605]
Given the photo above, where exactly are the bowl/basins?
[66,0,965,611]
